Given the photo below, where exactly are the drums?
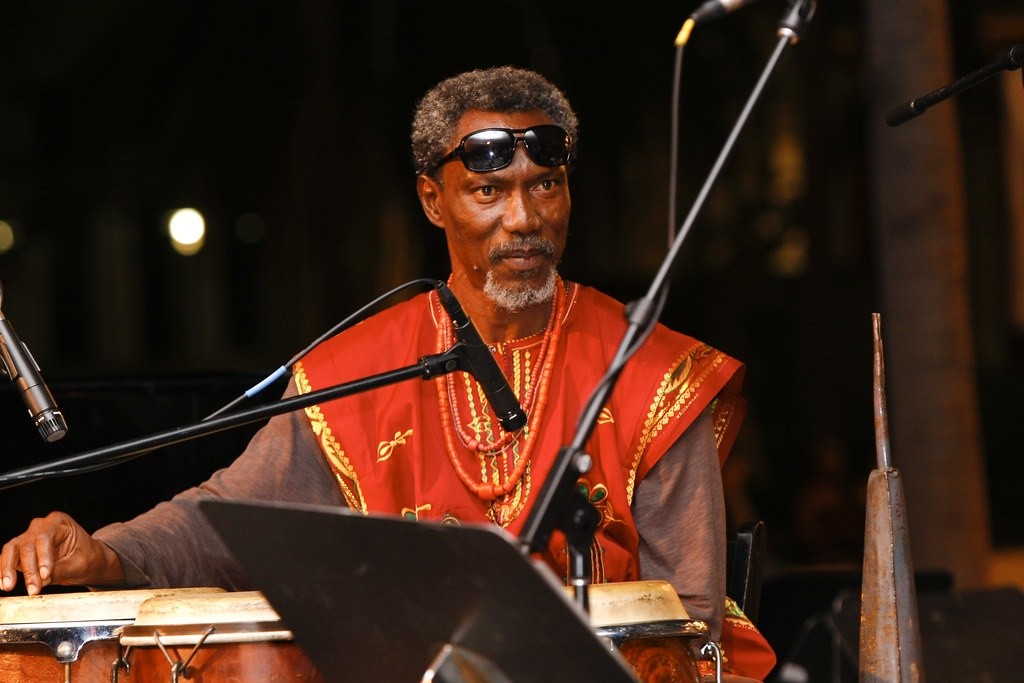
[558,577,713,683]
[117,589,324,683]
[0,585,227,683]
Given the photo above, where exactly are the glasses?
[429,125,572,176]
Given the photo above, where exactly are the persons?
[1,67,776,683]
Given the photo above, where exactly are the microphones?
[432,280,528,432]
[691,0,754,21]
[0,310,68,443]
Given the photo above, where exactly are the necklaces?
[446,272,558,456]
[437,272,567,501]
